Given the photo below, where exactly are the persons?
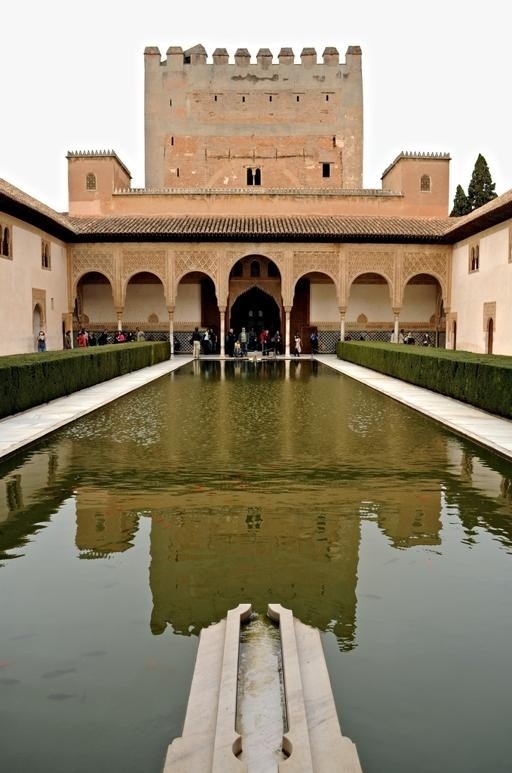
[189,359,319,383]
[359,336,365,341]
[421,331,431,346]
[390,328,415,345]
[36,330,49,351]
[157,333,181,352]
[345,333,353,341]
[191,326,304,357]
[309,328,319,355]
[66,327,155,349]
